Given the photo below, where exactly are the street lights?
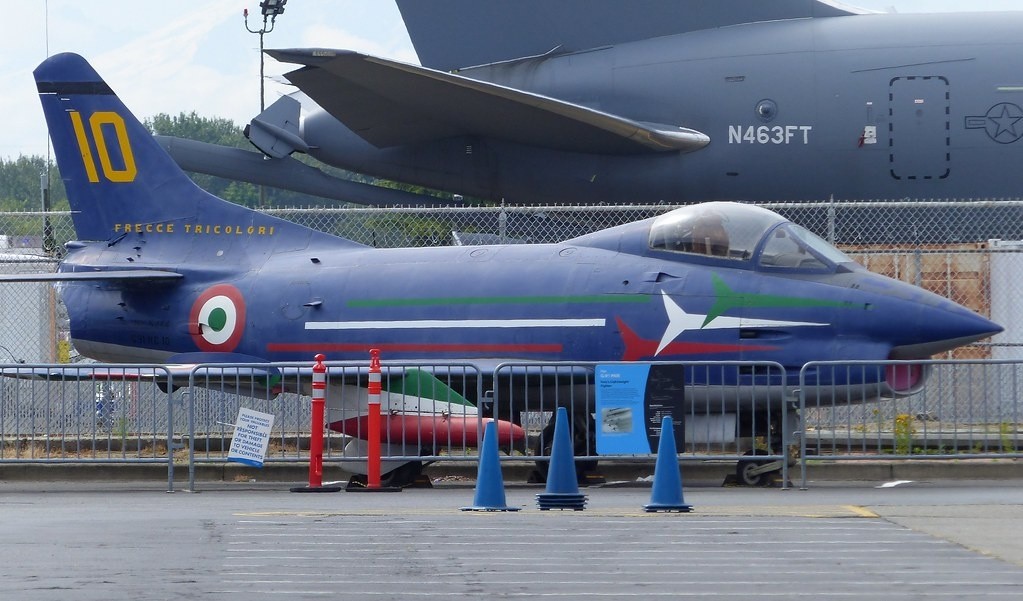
[244,0,288,209]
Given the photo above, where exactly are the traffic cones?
[456,421,522,511]
[533,407,590,512]
[643,416,695,513]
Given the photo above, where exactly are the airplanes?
[33,50,1006,449]
[242,0,1023,252]
